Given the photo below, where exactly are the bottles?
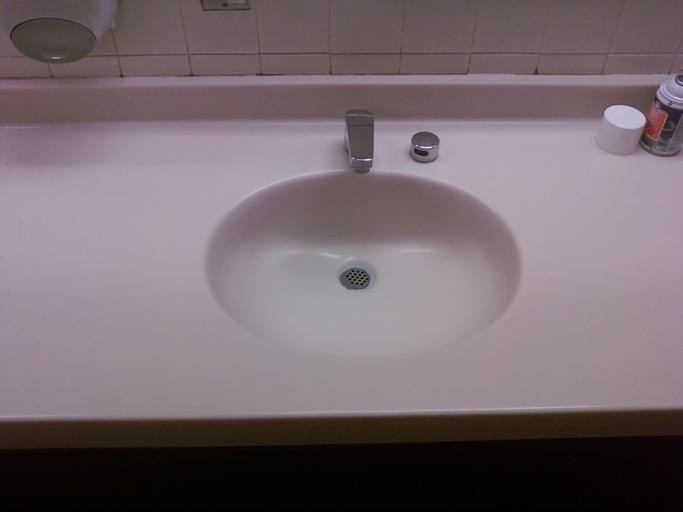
[639,75,683,157]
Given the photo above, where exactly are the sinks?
[206,167,523,359]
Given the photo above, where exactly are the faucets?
[344,107,374,174]
